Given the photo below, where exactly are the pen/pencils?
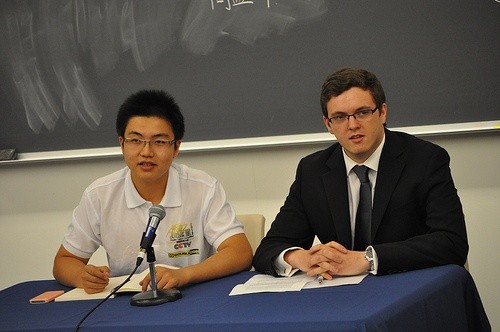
[318,275,323,286]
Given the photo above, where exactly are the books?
[55,264,181,302]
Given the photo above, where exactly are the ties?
[353,165,372,251]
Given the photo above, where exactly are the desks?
[0,265,492,332]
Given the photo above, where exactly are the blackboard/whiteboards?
[0,0,500,165]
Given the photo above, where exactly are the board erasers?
[0,149,16,160]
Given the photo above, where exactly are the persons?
[53,89,253,292]
[253,67,468,279]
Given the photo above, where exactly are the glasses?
[326,104,382,124]
[121,137,177,151]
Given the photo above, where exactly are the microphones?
[136,204,166,265]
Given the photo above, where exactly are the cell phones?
[30,291,64,304]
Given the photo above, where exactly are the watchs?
[364,249,374,271]
